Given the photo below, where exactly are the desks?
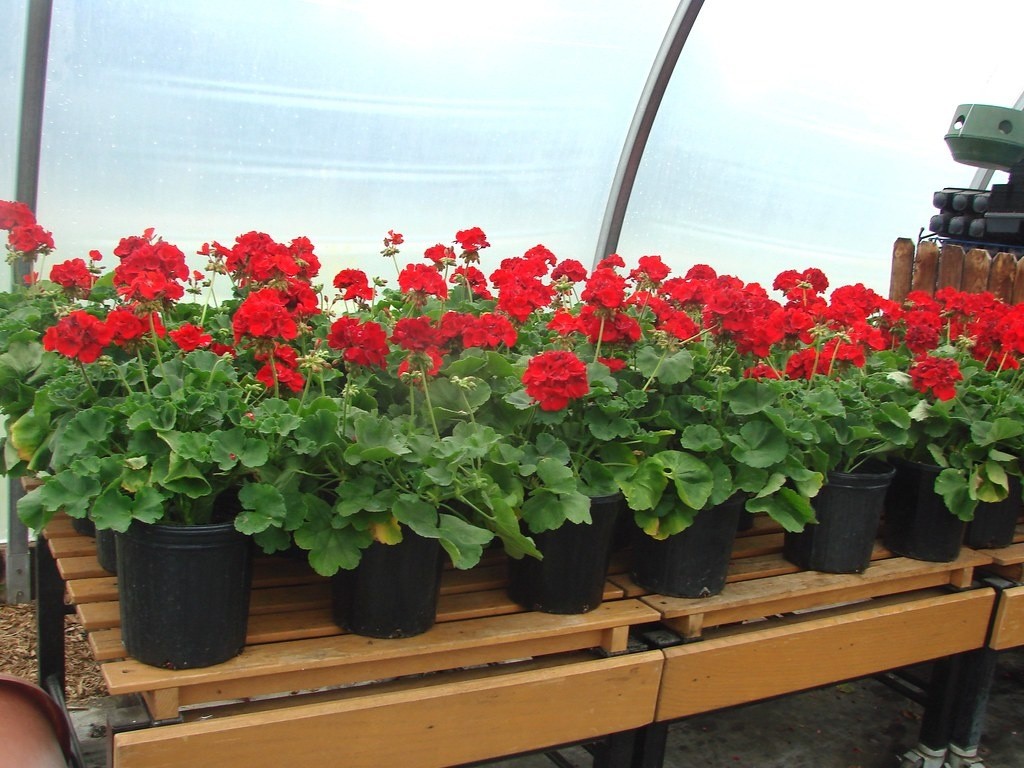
[18,475,1024,768]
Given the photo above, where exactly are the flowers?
[0,201,1023,574]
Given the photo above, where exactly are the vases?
[781,457,897,574]
[332,521,436,638]
[507,492,756,614]
[882,455,972,563]
[70,503,256,672]
[962,461,1020,550]
